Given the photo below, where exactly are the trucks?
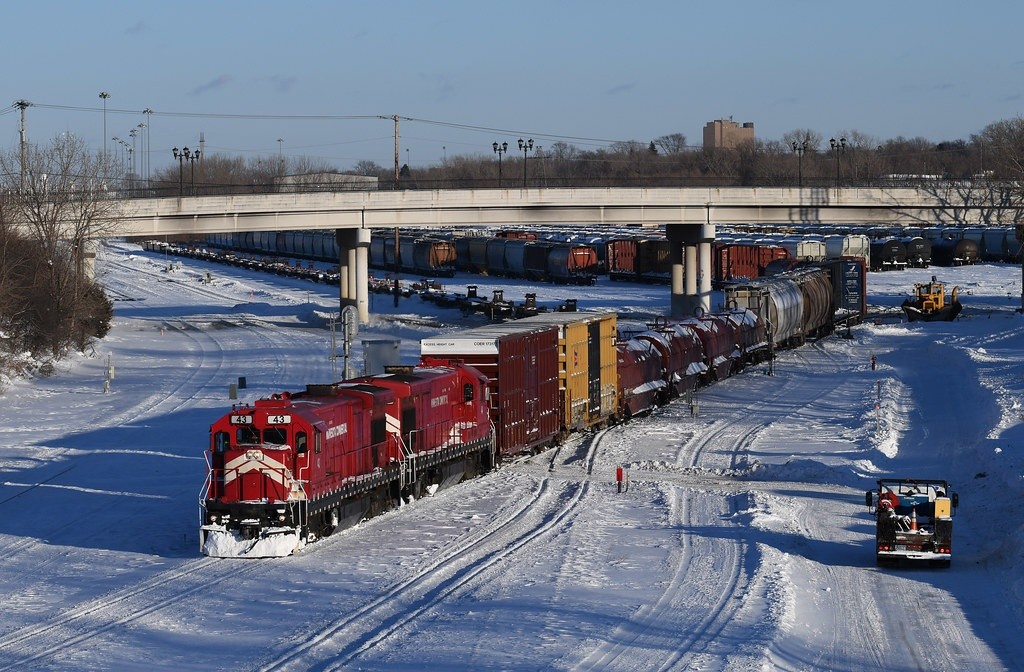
[865,479,959,568]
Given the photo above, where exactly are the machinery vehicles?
[900,276,962,323]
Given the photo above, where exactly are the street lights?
[136,122,147,179]
[186,148,200,195]
[99,92,111,159]
[277,136,284,161]
[792,141,808,186]
[171,146,190,196]
[406,148,409,167]
[830,136,846,186]
[517,138,534,187]
[443,146,446,161]
[492,141,508,187]
[142,107,152,190]
[112,129,139,187]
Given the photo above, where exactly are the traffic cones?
[910,508,918,531]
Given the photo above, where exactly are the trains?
[204,224,1024,286]
[198,266,835,558]
